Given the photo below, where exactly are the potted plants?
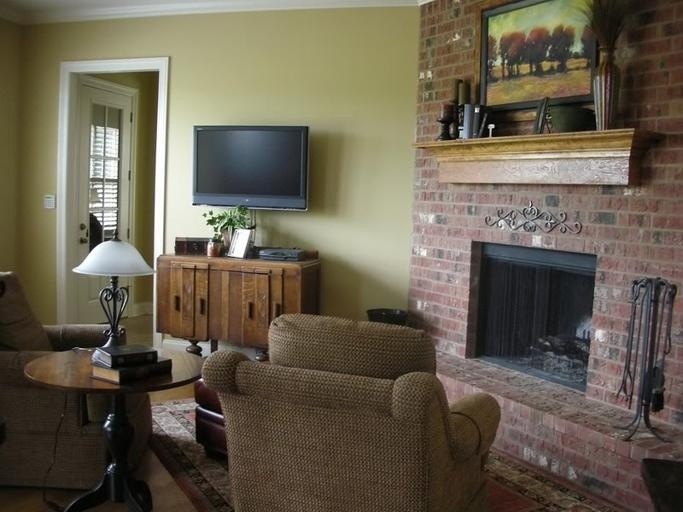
[583,0,627,131]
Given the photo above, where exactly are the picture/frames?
[226,229,252,258]
[474,0,598,115]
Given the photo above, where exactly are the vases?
[221,230,233,247]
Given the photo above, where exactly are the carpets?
[146,396,529,511]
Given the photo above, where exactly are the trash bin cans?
[367,307,408,325]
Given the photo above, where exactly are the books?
[88,356,172,385]
[472,104,487,137]
[93,344,157,368]
[479,112,491,137]
[463,102,472,139]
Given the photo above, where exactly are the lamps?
[71,230,157,347]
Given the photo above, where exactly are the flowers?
[205,205,253,231]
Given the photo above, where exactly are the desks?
[23,347,204,511]
[640,458,683,511]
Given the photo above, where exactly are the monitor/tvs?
[191,125,308,212]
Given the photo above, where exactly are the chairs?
[200,313,500,512]
[1,271,155,491]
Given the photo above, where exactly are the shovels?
[651,284,677,412]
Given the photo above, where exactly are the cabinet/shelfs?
[156,253,323,353]
